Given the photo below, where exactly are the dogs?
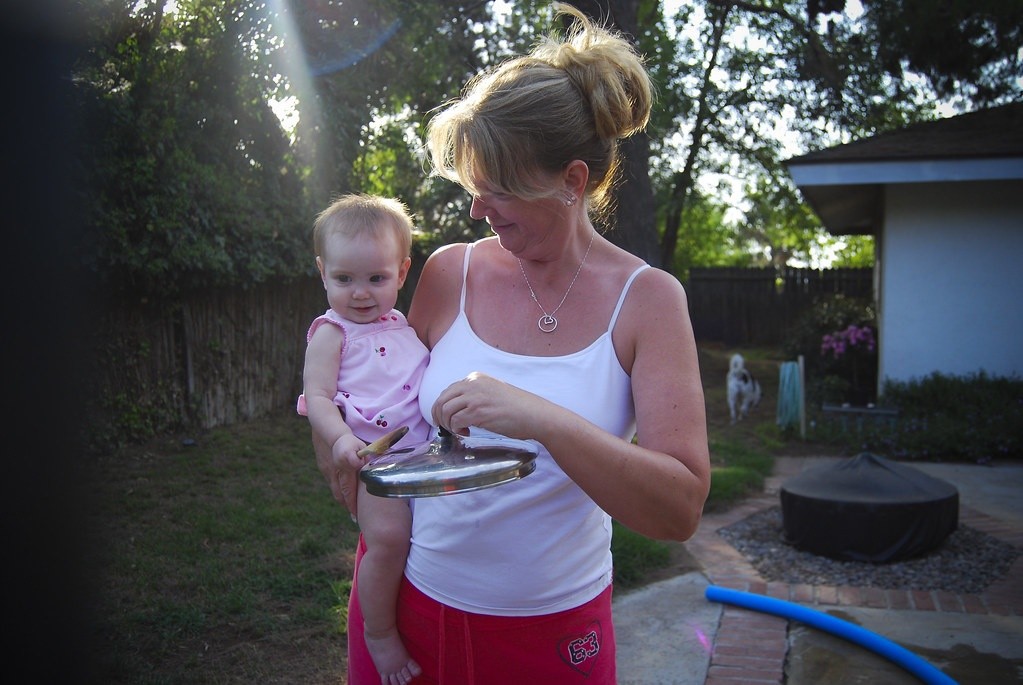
[726,353,762,425]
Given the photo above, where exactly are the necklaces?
[519,229,595,333]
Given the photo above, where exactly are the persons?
[310,27,714,685]
[296,195,433,685]
